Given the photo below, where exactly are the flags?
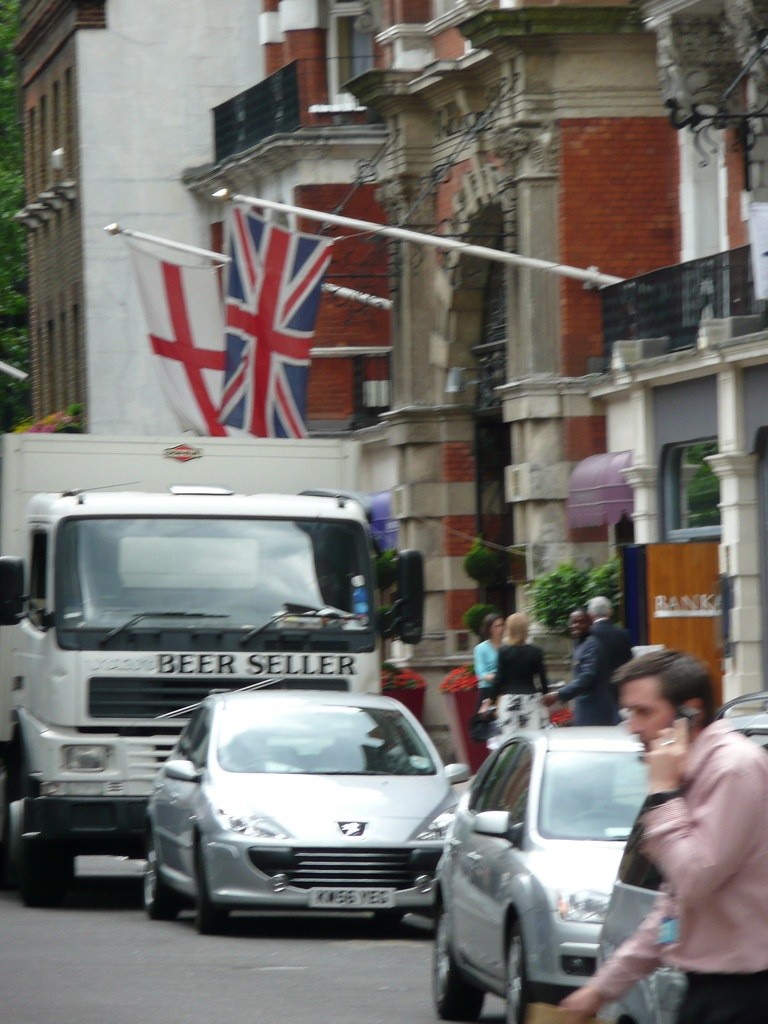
[130,245,226,437]
[218,206,335,440]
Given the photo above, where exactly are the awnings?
[564,450,635,529]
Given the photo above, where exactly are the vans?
[595,694,768,1024]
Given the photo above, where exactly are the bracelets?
[482,698,490,704]
[650,791,680,806]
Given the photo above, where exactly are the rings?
[663,739,675,745]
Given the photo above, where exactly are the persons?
[473,614,504,710]
[567,609,589,645]
[478,613,552,751]
[536,596,633,728]
[561,648,767,1024]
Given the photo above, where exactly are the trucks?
[0,432,424,901]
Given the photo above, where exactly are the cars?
[430,728,650,1024]
[142,689,471,935]
